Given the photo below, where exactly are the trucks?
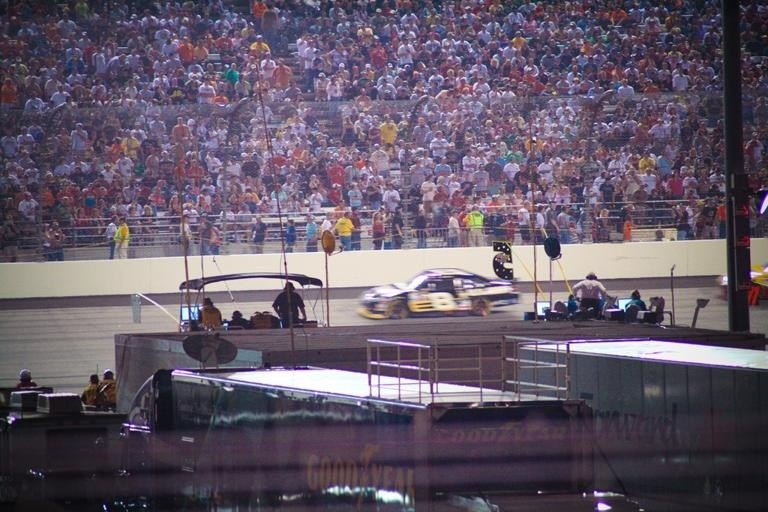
[120,364,613,511]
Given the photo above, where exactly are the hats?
[19,369,30,378]
[202,298,213,306]
[231,312,242,318]
[283,282,296,290]
[103,371,114,375]
[89,374,99,382]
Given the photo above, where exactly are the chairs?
[554,301,569,313]
[627,304,641,322]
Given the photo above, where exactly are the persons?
[81,374,108,411]
[625,289,647,311]
[199,298,222,327]
[17,369,36,388]
[272,282,306,328]
[572,272,613,319]
[1,1,768,265]
[101,368,117,413]
[228,310,248,331]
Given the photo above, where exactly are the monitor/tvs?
[618,298,632,313]
[181,306,199,321]
[534,301,551,316]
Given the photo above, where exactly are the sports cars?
[716,264,768,307]
[355,267,520,319]
